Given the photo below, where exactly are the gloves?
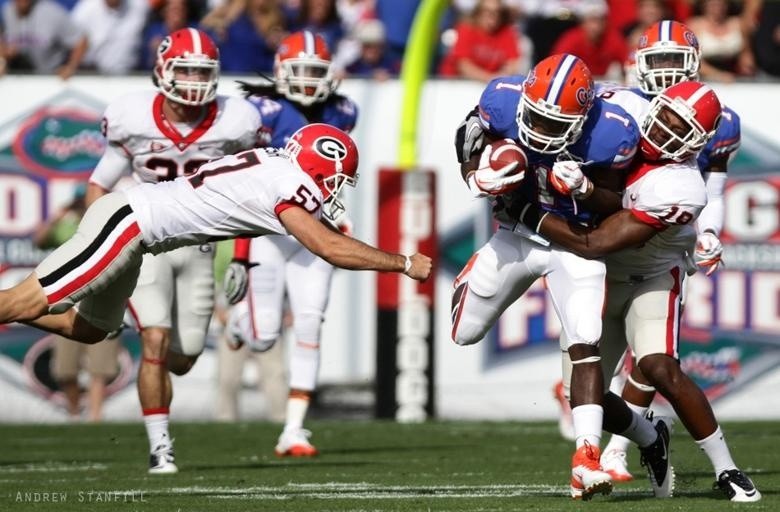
[549,160,595,201]
[223,256,248,306]
[492,190,551,234]
[694,231,725,276]
[467,144,526,198]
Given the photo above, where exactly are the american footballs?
[489,139,528,179]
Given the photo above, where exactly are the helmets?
[658,80,722,143]
[521,52,596,120]
[272,30,332,81]
[283,122,359,205]
[155,27,220,82]
[635,19,700,59]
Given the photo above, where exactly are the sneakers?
[568,444,615,502]
[552,381,580,443]
[599,447,634,482]
[637,416,676,499]
[273,430,320,457]
[147,444,179,475]
[106,319,131,341]
[715,469,762,504]
[224,301,250,351]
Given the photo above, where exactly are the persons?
[1,1,779,83]
[447,16,763,501]
[0,25,434,473]
